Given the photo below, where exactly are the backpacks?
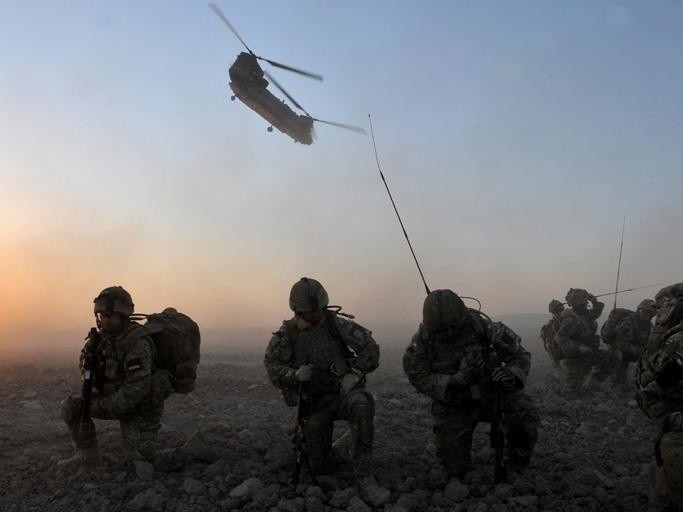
[634,284,683,420]
[601,308,641,346]
[121,307,200,394]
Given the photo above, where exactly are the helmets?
[549,288,591,313]
[289,277,329,312]
[94,286,135,316]
[637,299,657,316]
[423,289,467,334]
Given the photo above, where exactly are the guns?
[290,347,338,492]
[483,340,511,484]
[77,327,100,443]
[569,335,611,365]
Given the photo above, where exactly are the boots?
[331,429,352,460]
[176,431,220,460]
[354,461,391,509]
[57,445,100,467]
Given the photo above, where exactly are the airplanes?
[209,2,368,146]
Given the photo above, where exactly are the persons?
[631,281,683,512]
[604,298,657,384]
[545,287,624,399]
[401,288,540,478]
[264,277,381,467]
[55,284,172,468]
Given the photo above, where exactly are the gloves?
[491,365,517,390]
[577,344,593,354]
[295,366,313,383]
[339,372,360,397]
[454,352,486,387]
